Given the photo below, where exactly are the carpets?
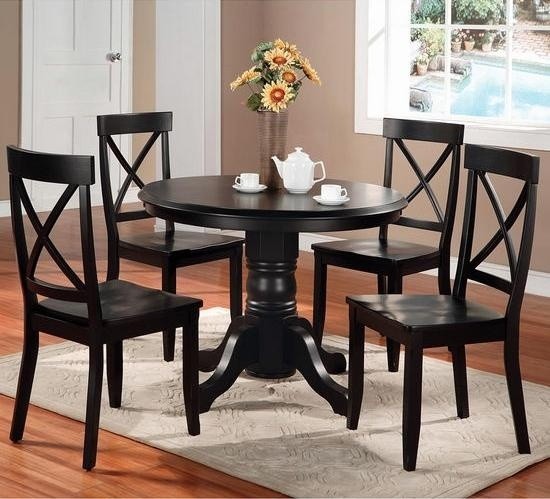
[0,306,550,498]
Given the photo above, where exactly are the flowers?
[477,30,492,43]
[230,38,321,112]
[416,44,427,63]
[451,26,466,41]
[462,29,476,43]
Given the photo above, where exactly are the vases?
[464,40,475,51]
[451,38,462,53]
[482,41,492,52]
[417,63,428,74]
[256,109,289,190]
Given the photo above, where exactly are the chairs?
[96,112,246,362]
[6,145,202,473]
[310,117,465,372]
[345,141,539,471]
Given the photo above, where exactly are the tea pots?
[271,147,326,194]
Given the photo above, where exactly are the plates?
[312,195,350,205]
[231,186,268,192]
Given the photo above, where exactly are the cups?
[320,185,347,197]
[236,172,259,187]
